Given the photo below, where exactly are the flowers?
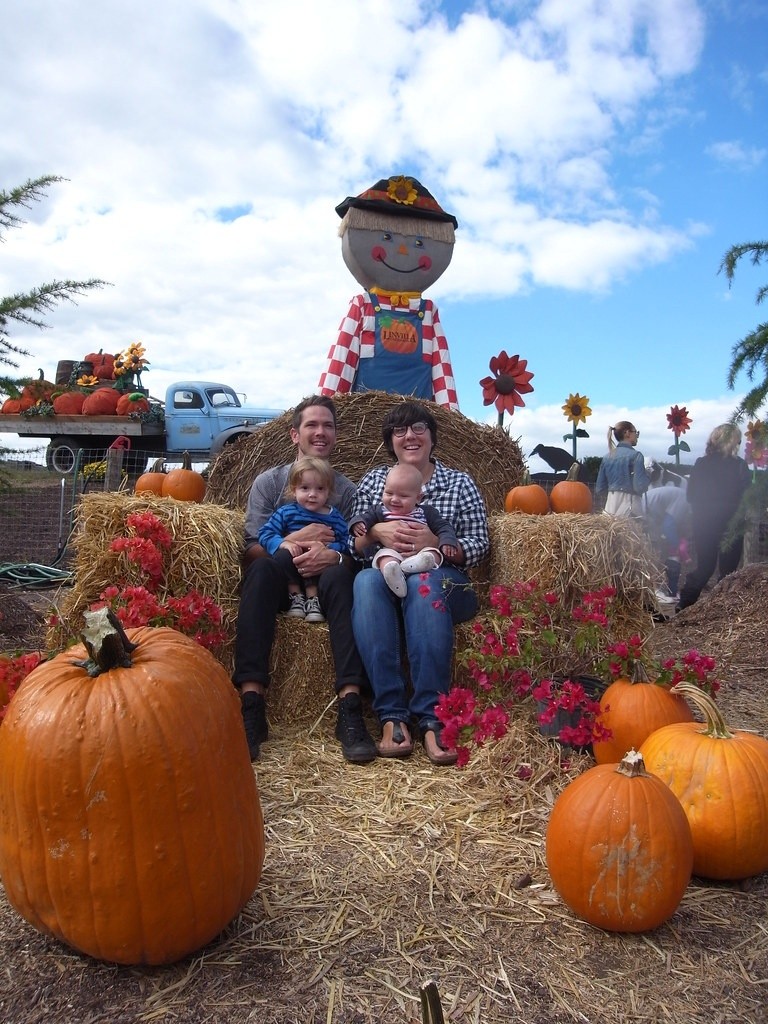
[113,343,151,390]
[417,570,722,769]
[77,375,99,387]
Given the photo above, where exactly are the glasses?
[392,419,429,437]
[630,430,639,437]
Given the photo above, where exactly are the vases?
[531,675,610,747]
[121,388,149,399]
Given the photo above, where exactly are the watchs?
[336,551,343,565]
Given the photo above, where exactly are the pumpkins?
[161,451,206,503]
[549,463,593,514]
[0,602,264,969]
[1,349,149,416]
[503,469,549,515]
[545,748,693,932]
[592,659,694,766]
[133,458,168,498]
[634,680,768,881]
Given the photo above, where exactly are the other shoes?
[671,593,681,600]
[378,716,413,757]
[643,604,669,623]
[416,719,459,765]
[675,602,689,614]
[654,590,675,603]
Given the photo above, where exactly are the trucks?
[0,381,287,475]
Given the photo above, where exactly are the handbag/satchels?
[626,491,649,533]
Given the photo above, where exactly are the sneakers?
[336,692,378,761]
[304,595,325,623]
[239,691,268,761]
[288,592,306,618]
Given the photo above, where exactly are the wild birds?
[528,443,583,474]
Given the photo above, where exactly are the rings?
[412,544,415,551]
[302,568,305,573]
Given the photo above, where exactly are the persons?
[315,176,459,414]
[231,396,376,764]
[674,424,751,612]
[643,487,689,603]
[596,420,671,624]
[347,402,491,765]
